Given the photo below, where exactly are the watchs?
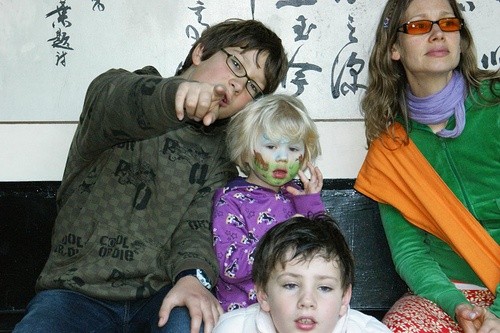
[174,267,212,290]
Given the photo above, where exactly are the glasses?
[220,48,265,101]
[398,16,464,34]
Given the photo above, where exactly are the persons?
[214,94,325,313]
[212,211,390,333]
[10,17,288,333]
[354,0,500,333]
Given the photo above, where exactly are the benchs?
[0,175,411,333]
